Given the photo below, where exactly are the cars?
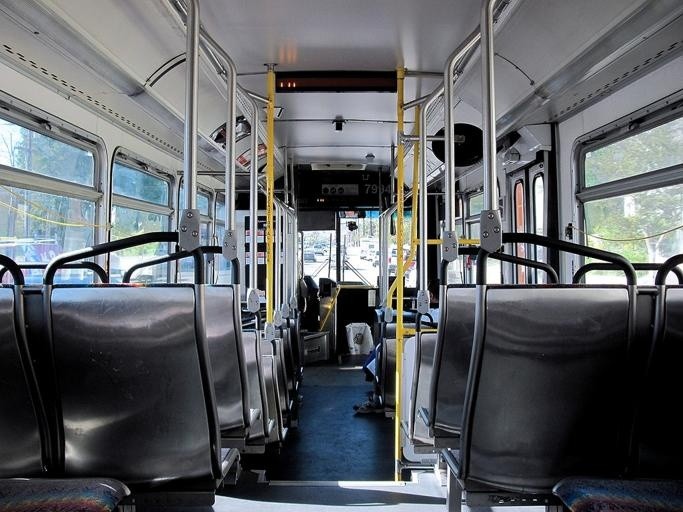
[303,243,330,262]
[360,248,416,267]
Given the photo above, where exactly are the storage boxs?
[304,329,331,363]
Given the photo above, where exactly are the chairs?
[367,247,680,462]
[441,233,682,510]
[3,245,304,462]
[1,232,246,512]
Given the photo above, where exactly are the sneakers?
[351,391,382,414]
